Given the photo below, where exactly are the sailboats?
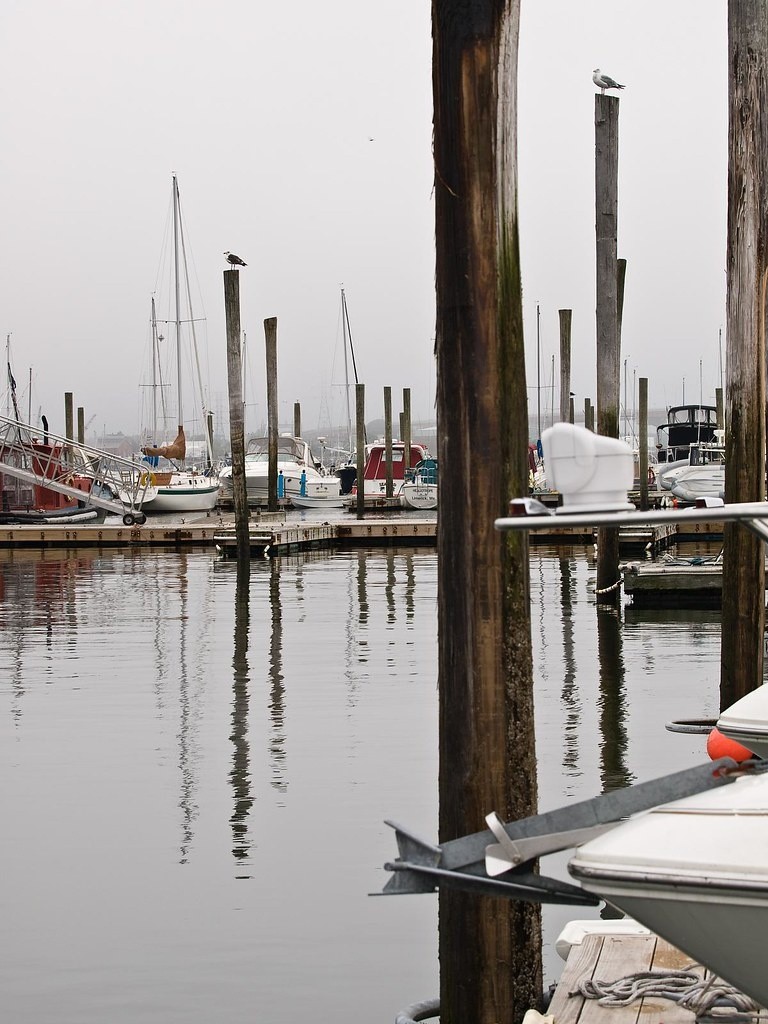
[528,304,725,501]
[0,171,437,523]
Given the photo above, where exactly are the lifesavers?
[64,475,75,502]
[140,471,157,486]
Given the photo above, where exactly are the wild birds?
[592,69,626,94]
[222,250,248,270]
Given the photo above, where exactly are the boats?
[624,553,768,594]
[709,672,768,779]
[364,756,768,1009]
